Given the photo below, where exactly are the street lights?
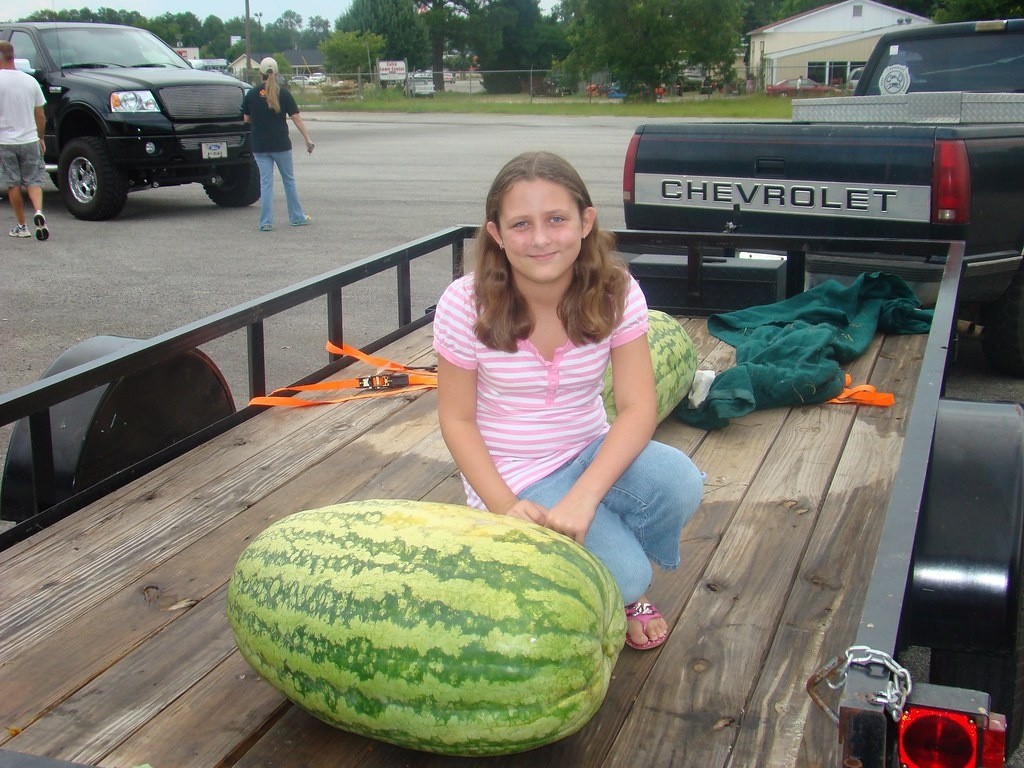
[255,11,264,52]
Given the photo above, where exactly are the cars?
[444,73,455,83]
[408,70,433,78]
[288,75,309,85]
[307,73,326,85]
[848,66,865,94]
[334,80,344,87]
[674,72,725,94]
[764,78,842,98]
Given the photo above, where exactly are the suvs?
[0,23,263,220]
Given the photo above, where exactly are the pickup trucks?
[402,79,436,98]
[624,11,1024,383]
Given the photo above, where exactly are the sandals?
[626,602,669,650]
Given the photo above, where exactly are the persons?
[431,151,705,648]
[241,56,315,232]
[797,75,805,97]
[0,39,49,241]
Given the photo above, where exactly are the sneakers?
[32,210,50,241]
[9,223,32,237]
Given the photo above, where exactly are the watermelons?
[602,310,698,426]
[226,498,627,755]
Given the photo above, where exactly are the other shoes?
[262,225,271,231]
[292,214,312,226]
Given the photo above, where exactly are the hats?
[259,56,278,73]
